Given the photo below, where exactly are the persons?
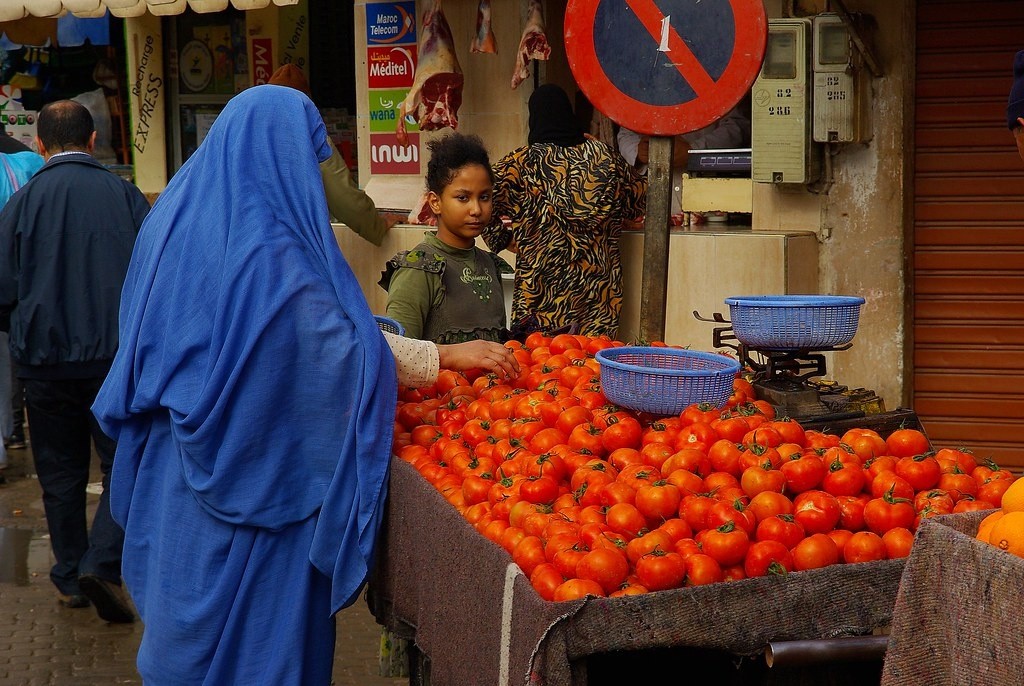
[91,84,523,686]
[478,84,648,340]
[617,110,750,216]
[377,133,514,344]
[0,101,154,617]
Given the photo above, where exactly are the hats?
[268,63,311,98]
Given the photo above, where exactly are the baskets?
[724,294,866,349]
[595,345,740,417]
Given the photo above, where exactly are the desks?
[365,341,908,686]
[882,510,1024,686]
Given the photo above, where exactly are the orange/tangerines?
[974,476,1024,563]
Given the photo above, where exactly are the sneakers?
[5,434,27,450]
[77,573,134,625]
[56,589,90,608]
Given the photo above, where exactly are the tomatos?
[393,331,1012,603]
